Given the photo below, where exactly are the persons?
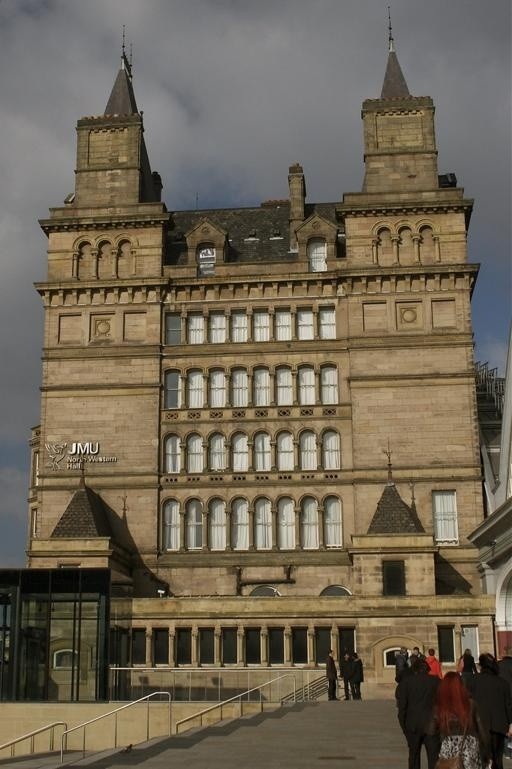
[395,646,512,769]
[338,652,358,700]
[349,653,364,699]
[325,650,341,700]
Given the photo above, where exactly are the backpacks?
[457,658,464,672]
[432,756,465,769]
